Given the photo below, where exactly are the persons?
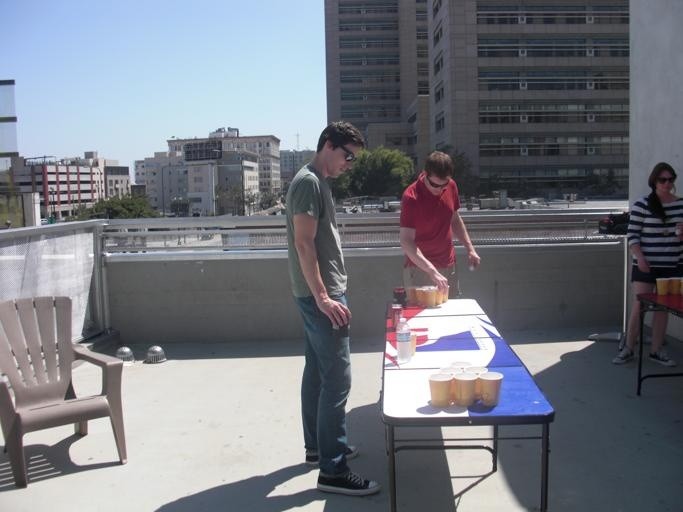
[399,149,482,298]
[285,118,382,498]
[611,162,683,366]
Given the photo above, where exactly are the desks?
[379,298,555,512]
[636,291,683,396]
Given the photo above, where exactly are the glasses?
[656,178,676,183]
[339,143,356,161]
[426,176,449,189]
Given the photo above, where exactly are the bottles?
[395,287,404,296]
[391,307,402,328]
[396,317,410,361]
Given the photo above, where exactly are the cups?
[333,321,350,337]
[410,332,416,356]
[656,278,683,296]
[408,285,449,307]
[429,362,503,408]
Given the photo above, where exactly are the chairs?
[0,295,128,487]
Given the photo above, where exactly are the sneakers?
[317,472,381,496]
[306,445,358,464]
[613,345,636,364]
[648,349,677,367]
